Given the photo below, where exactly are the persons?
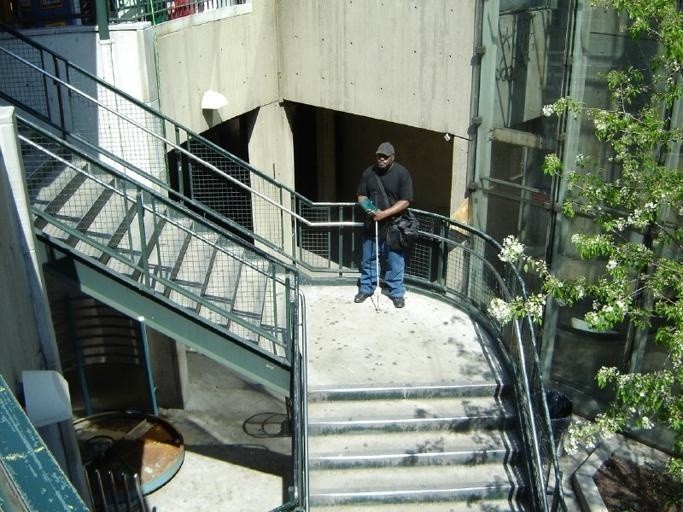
[354,142,414,308]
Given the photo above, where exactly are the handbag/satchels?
[386,209,421,253]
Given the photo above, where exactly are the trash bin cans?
[531,389,574,466]
[139,0,169,26]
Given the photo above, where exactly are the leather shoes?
[354,290,374,303]
[393,295,405,309]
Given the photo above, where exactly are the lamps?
[17,370,74,429]
[201,89,228,109]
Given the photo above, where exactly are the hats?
[376,141,396,157]
